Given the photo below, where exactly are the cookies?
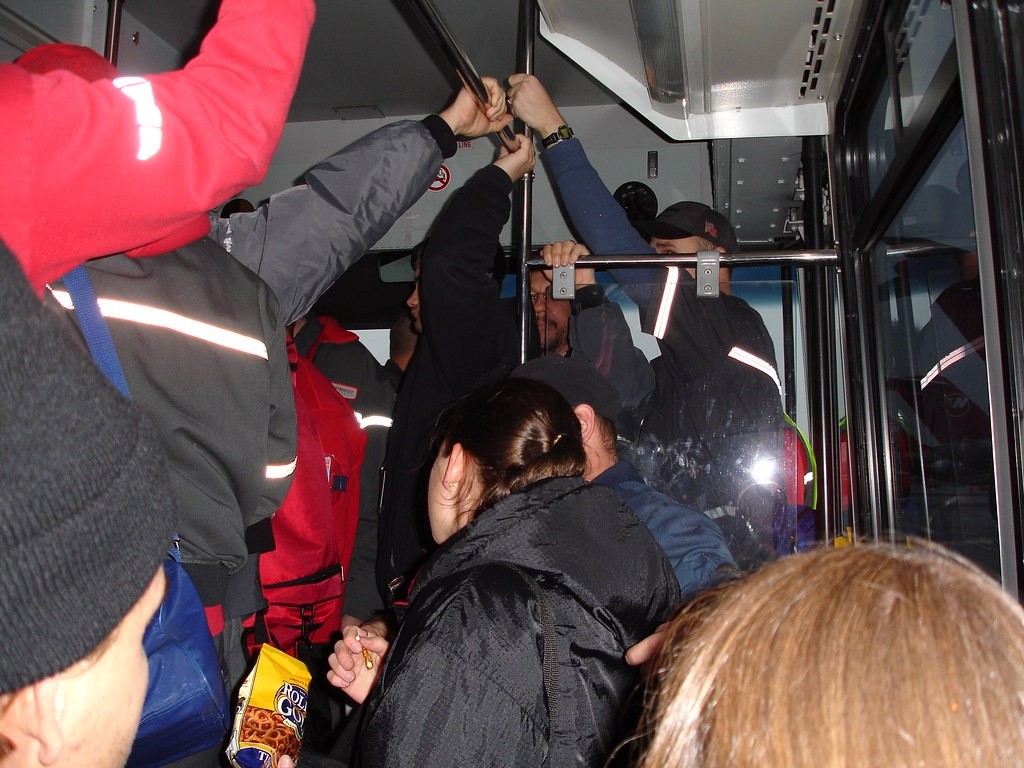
[241,707,301,767]
[356,635,373,670]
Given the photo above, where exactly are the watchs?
[542,124,575,148]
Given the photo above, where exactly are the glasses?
[530,292,553,305]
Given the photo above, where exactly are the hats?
[635,201,735,247]
[507,354,625,430]
[0,237,177,696]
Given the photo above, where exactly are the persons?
[1,0,317,301]
[208,72,791,767]
[1,238,184,768]
[14,38,298,681]
[644,535,1023,765]
[912,209,1010,482]
[328,371,679,767]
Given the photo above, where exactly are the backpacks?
[242,335,369,665]
